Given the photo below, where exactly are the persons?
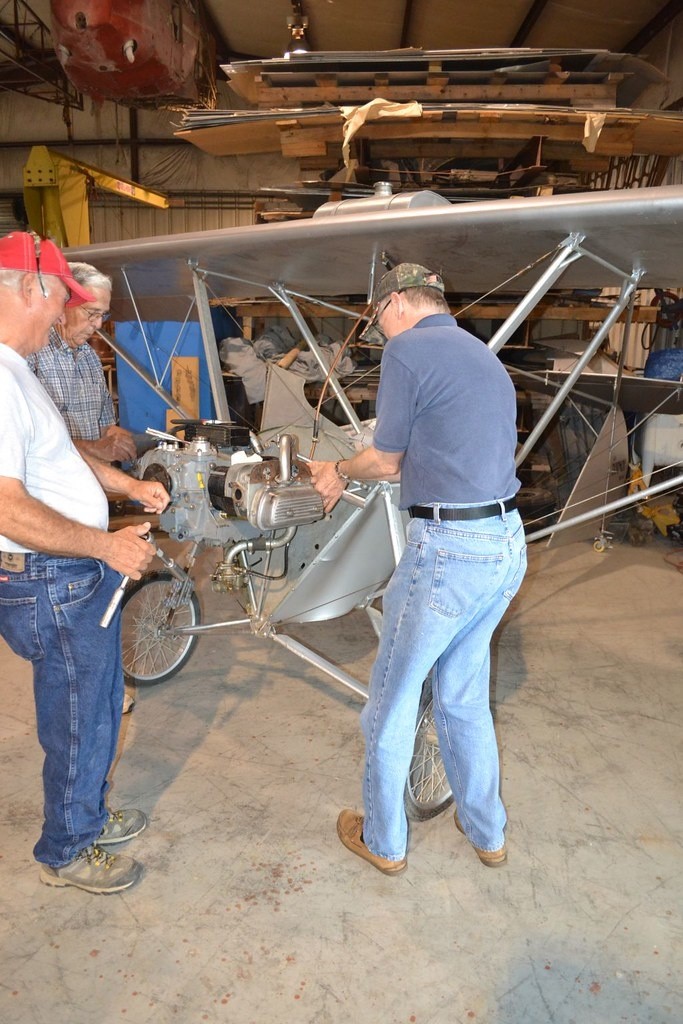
[305,263,527,876]
[0,233,170,893]
[26,262,137,713]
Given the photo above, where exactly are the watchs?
[335,459,352,482]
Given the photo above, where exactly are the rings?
[325,499,329,504]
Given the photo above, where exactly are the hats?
[359,262,445,346]
[0,230,96,308]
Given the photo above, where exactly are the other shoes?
[453,807,508,868]
[336,809,408,877]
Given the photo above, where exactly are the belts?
[408,495,517,521]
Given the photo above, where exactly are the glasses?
[371,289,406,335]
[26,223,51,299]
[76,305,111,323]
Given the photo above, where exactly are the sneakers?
[121,693,135,714]
[39,842,142,895]
[92,808,147,846]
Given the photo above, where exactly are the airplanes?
[57,181,683,820]
